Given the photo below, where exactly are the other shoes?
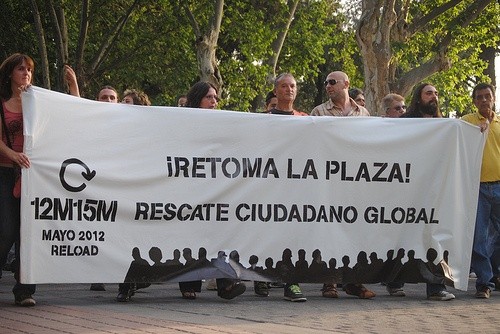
[182,288,197,298]
[90,286,105,291]
[14,296,37,306]
[116,289,132,301]
[220,283,246,299]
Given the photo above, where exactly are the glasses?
[205,95,220,102]
[393,105,408,110]
[325,79,344,86]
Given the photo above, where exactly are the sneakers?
[254,281,269,297]
[428,290,456,300]
[320,283,337,298]
[387,288,406,296]
[283,283,307,301]
[349,284,375,299]
[475,284,491,299]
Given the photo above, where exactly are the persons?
[0,52,36,307]
[461,82,500,298]
[6,65,407,303]
[386,83,458,301]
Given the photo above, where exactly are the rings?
[20,160,22,162]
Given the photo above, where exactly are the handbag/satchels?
[0,163,20,198]
